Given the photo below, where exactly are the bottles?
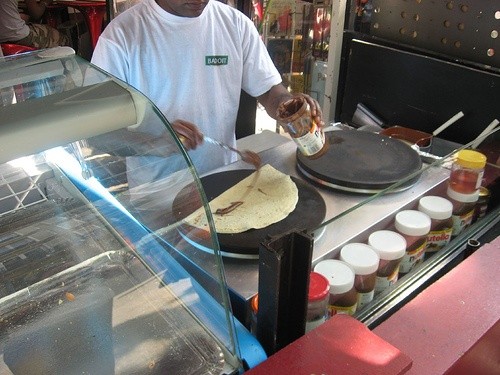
[446,186,479,240]
[393,210,431,278]
[313,259,359,319]
[418,196,453,261]
[305,271,330,333]
[338,242,379,311]
[471,186,490,223]
[368,229,406,294]
[450,149,487,195]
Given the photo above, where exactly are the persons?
[0,0,73,74]
[82,0,324,189]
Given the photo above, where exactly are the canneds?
[275,95,329,160]
[251,210,431,338]
[418,148,489,264]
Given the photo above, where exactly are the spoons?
[201,135,261,166]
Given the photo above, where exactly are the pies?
[183,163,299,234]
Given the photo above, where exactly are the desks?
[48,0,107,51]
